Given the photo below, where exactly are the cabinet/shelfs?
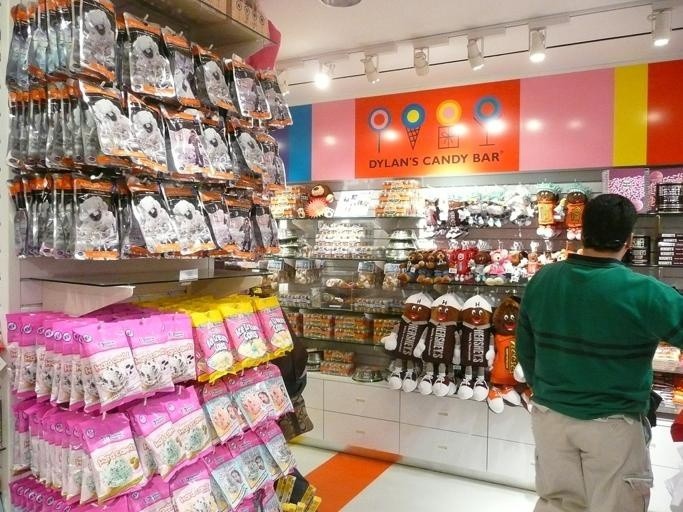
[303,371,683,512]
[273,216,683,347]
[22,0,273,288]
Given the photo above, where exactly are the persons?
[515,194,683,511]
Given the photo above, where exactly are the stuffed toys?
[380,185,586,413]
[297,184,335,219]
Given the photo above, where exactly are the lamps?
[278,0,675,95]
